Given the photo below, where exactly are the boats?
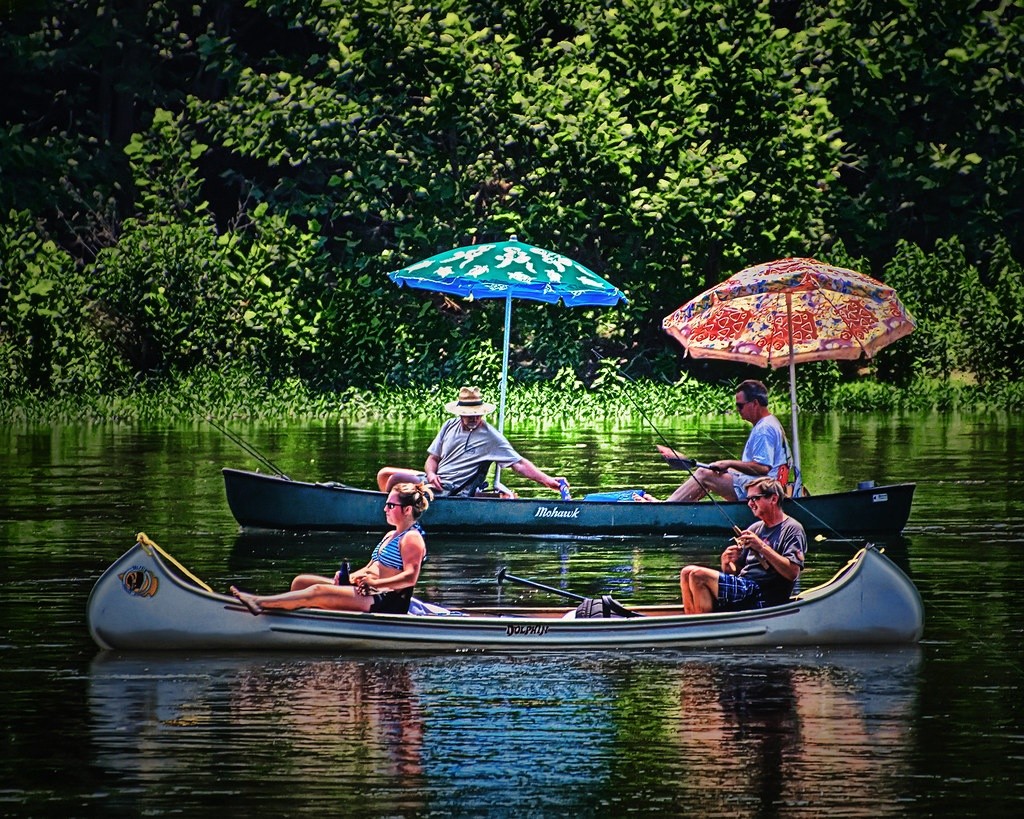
[219,467,918,541]
[84,531,928,655]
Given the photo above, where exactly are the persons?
[680,477,807,614]
[632,380,792,501]
[230,483,433,616]
[377,387,570,494]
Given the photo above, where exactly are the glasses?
[384,502,405,509]
[746,495,767,503]
[736,400,753,408]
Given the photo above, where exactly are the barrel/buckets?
[858,480,874,489]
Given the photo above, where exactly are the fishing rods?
[593,348,772,570]
[62,298,290,481]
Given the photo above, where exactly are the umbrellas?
[386,235,633,493]
[663,257,917,498]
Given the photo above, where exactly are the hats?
[446,386,496,416]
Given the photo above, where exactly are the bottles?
[558,479,572,501]
[337,556,353,585]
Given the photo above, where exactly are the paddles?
[496,567,588,601]
[656,444,723,471]
